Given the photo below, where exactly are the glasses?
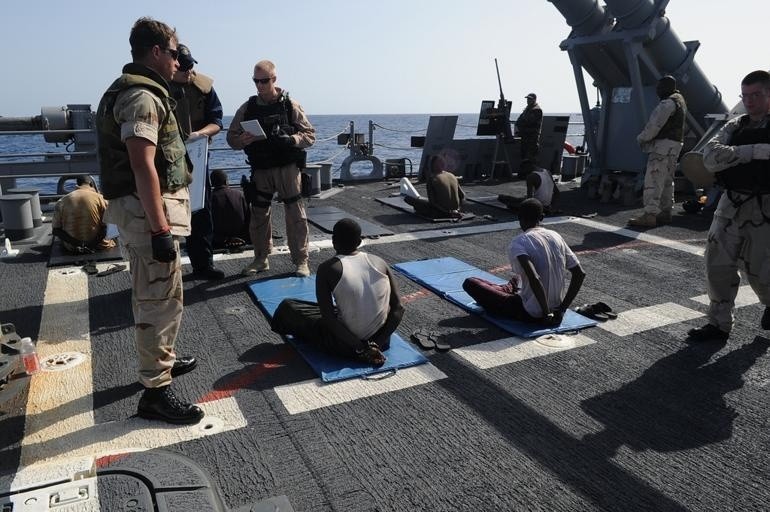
[739,92,767,99]
[252,77,273,84]
[177,64,194,72]
[163,48,179,61]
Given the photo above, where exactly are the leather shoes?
[193,266,225,280]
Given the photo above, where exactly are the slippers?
[575,301,617,322]
[98,263,126,277]
[272,229,282,238]
[412,327,453,351]
[84,262,98,274]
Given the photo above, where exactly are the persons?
[50,174,115,254]
[270,218,405,370]
[225,59,315,278]
[498,158,559,209]
[96,16,205,426]
[687,70,770,340]
[162,44,223,281]
[627,75,688,226]
[513,92,544,180]
[461,197,587,331]
[403,155,466,218]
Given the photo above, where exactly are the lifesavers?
[564,141,576,153]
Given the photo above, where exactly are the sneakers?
[761,307,770,330]
[688,323,729,338]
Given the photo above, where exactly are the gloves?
[150,226,177,262]
[750,143,769,158]
[273,130,295,150]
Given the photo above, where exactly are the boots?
[294,256,310,277]
[242,255,269,276]
[627,209,671,228]
[138,384,204,424]
[172,356,197,375]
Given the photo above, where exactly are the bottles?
[20,336,41,375]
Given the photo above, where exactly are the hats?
[525,94,537,100]
[177,44,198,72]
